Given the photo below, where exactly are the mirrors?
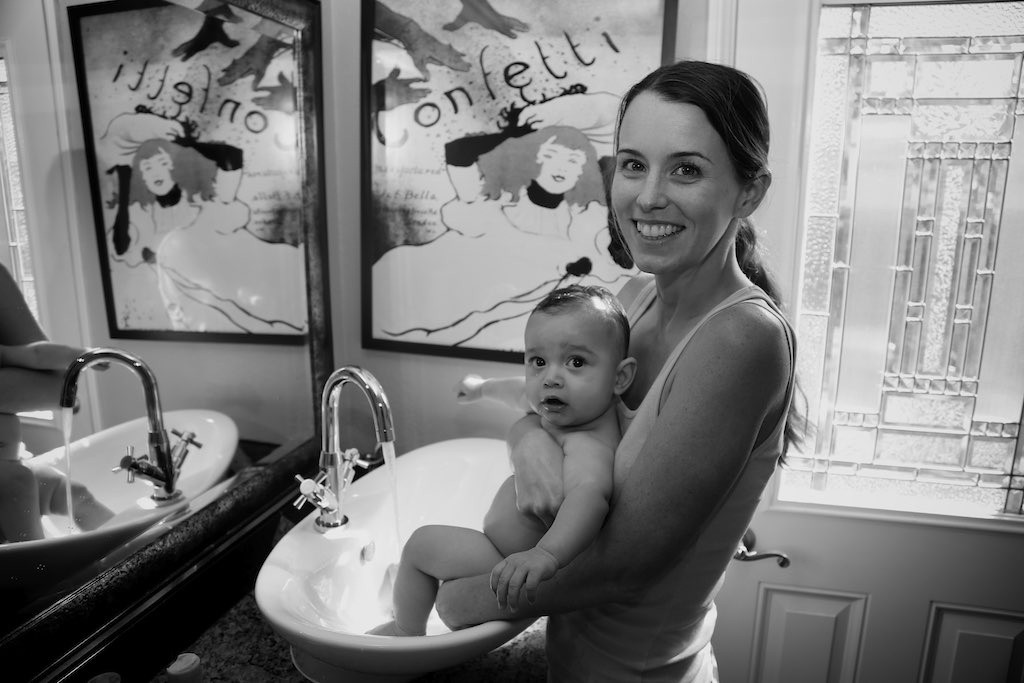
[0,0,337,641]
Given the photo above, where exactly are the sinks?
[252,432,539,681]
[0,409,242,570]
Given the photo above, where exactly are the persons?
[362,286,637,639]
[0,266,116,544]
[434,64,811,683]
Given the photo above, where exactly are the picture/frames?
[66,0,309,345]
[360,0,679,364]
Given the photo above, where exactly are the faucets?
[291,362,400,530]
[59,347,206,507]
